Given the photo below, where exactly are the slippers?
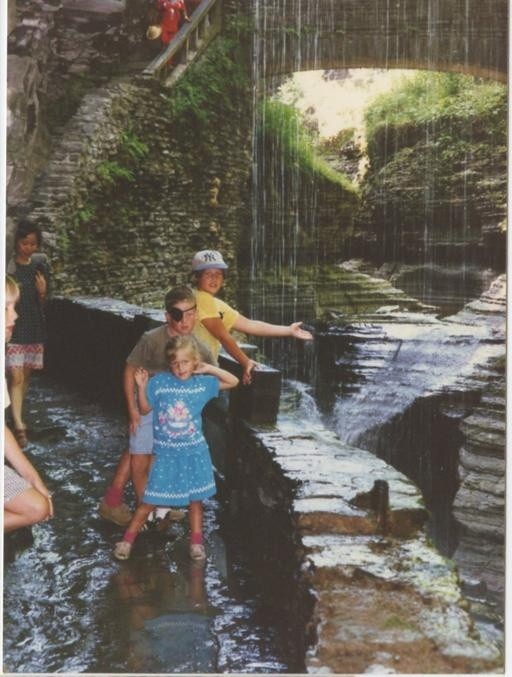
[14,426,29,448]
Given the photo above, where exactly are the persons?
[114,332,240,562]
[97,285,221,526]
[3,270,56,537]
[154,1,190,43]
[4,217,53,450]
[189,247,313,387]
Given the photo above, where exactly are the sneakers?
[96,499,135,526]
[113,540,133,561]
[187,543,208,562]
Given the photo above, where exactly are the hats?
[191,249,229,271]
